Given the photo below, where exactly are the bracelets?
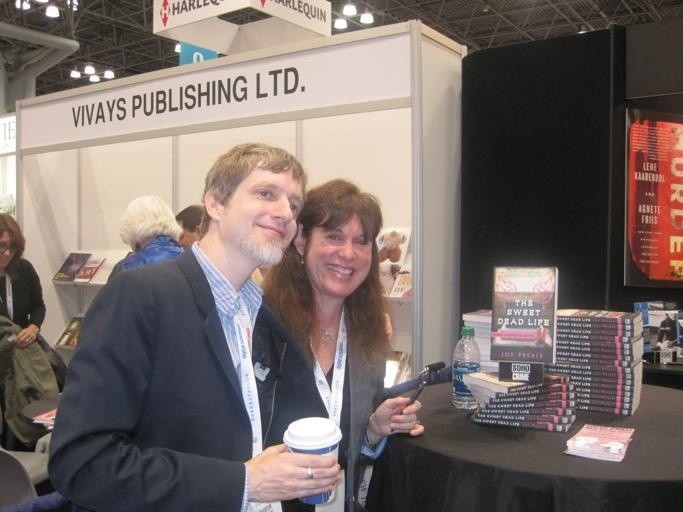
[365,428,373,446]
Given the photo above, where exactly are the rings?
[25,338,29,343]
[304,466,314,479]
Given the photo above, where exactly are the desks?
[370,381,682,511]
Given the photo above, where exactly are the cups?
[282,416,343,505]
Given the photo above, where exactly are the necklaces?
[313,316,337,343]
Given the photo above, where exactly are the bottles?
[450,326,483,410]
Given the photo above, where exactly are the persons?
[0,214,46,450]
[107,193,184,282]
[261,179,427,512]
[46,142,393,512]
[175,205,205,251]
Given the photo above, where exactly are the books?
[32,410,56,431]
[56,315,84,347]
[634,301,683,365]
[382,348,412,389]
[462,266,643,463]
[53,252,91,280]
[75,257,107,282]
[374,226,412,299]
